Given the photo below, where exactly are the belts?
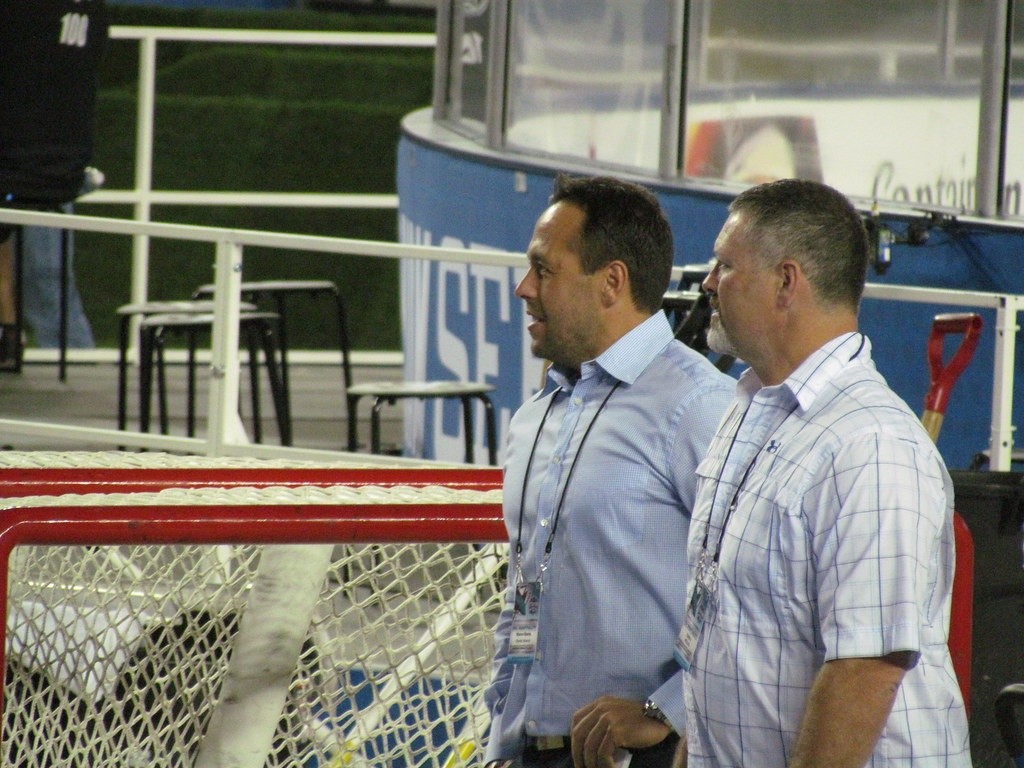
[520,731,680,760]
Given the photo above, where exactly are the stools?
[114,281,498,470]
[0,201,69,381]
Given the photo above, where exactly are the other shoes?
[0,323,25,372]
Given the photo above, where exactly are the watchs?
[644,700,675,731]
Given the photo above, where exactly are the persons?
[672,180,973,768]
[484,174,738,768]
[1,0,108,347]
